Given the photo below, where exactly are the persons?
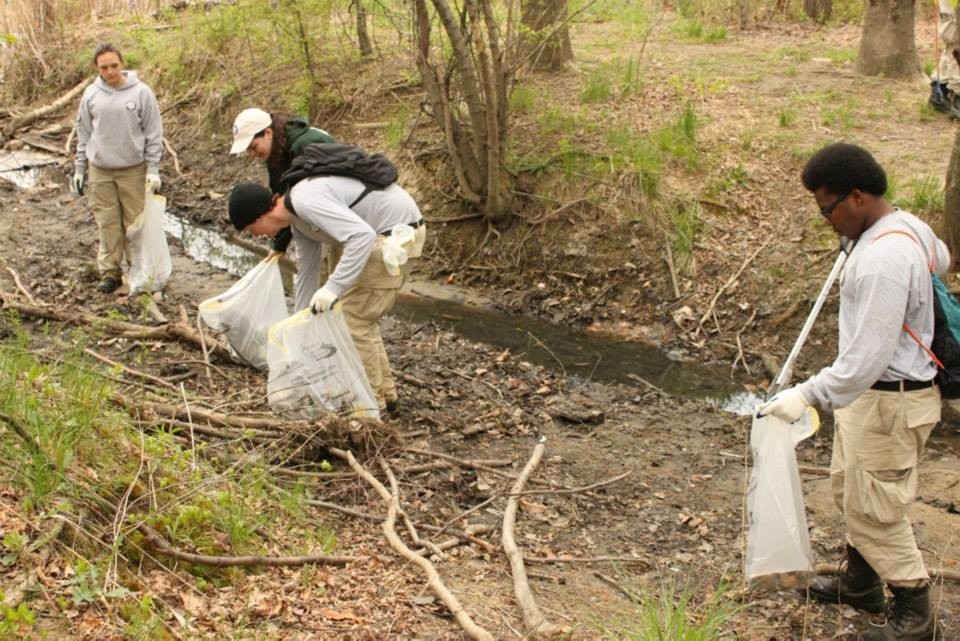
[756,142,955,641]
[230,108,337,312]
[72,44,161,292]
[229,175,425,420]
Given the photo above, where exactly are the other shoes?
[387,398,402,422]
[380,409,393,424]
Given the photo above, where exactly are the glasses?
[821,191,850,221]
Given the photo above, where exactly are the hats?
[230,107,272,153]
[229,183,272,231]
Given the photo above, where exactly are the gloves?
[73,171,85,197]
[755,385,808,424]
[309,286,340,316]
[145,173,162,192]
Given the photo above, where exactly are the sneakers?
[97,272,124,293]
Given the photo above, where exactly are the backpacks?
[278,142,400,218]
[871,229,960,399]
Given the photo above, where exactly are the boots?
[856,583,935,641]
[800,543,885,613]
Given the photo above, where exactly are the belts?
[870,377,939,392]
[381,217,425,236]
